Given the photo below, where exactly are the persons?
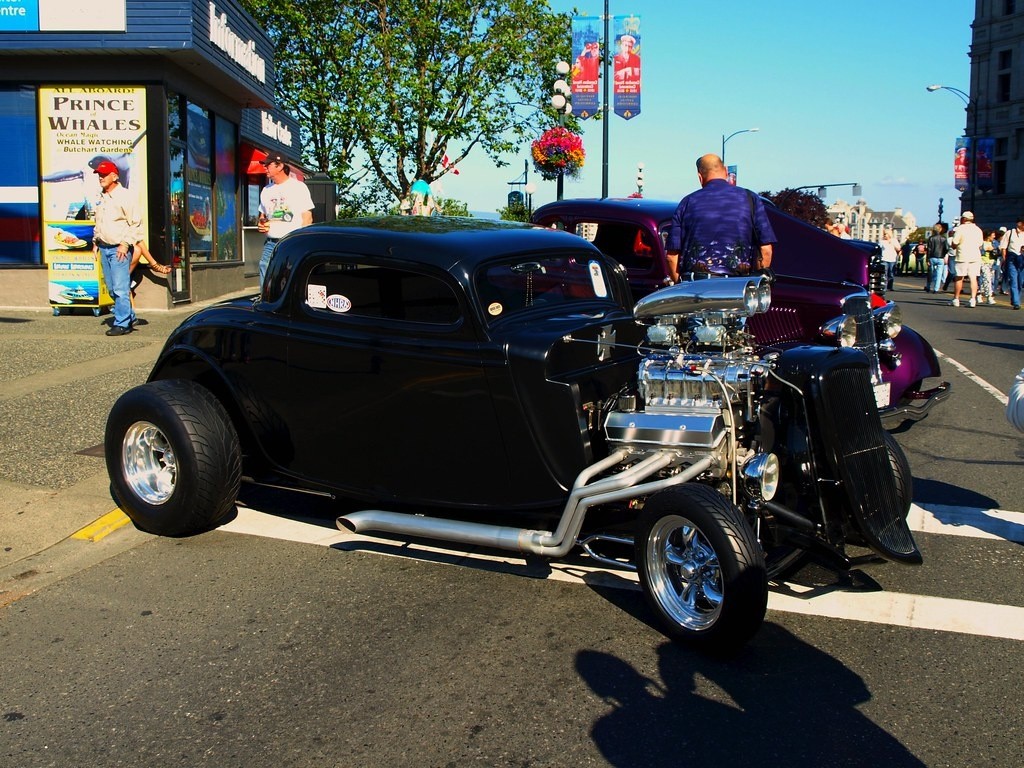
[255,152,315,295]
[665,154,777,284]
[825,210,1024,311]
[91,161,171,336]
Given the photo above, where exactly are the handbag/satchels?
[1001,249,1009,275]
[988,249,999,261]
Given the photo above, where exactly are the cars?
[530,198,950,431]
[103,216,926,658]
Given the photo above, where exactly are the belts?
[105,244,121,249]
[266,238,279,244]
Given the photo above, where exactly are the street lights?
[551,62,572,201]
[722,128,758,164]
[927,85,977,215]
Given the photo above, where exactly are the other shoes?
[131,318,139,327]
[933,290,938,294]
[986,297,996,304]
[105,326,133,336]
[887,287,895,292]
[924,287,930,292]
[938,288,947,292]
[977,294,983,304]
[960,290,964,294]
[969,297,976,308]
[952,299,959,307]
[999,291,1008,295]
[1014,305,1020,310]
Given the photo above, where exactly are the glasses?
[264,163,278,168]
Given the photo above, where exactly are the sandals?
[149,262,172,274]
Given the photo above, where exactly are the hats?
[999,226,1007,233]
[258,151,291,168]
[963,211,974,221]
[93,162,120,176]
